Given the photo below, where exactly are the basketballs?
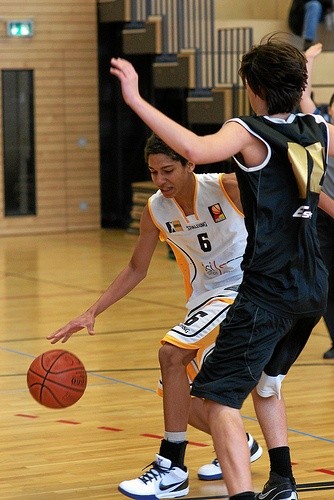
[26,347,88,409]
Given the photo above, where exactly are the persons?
[288,0,334,53]
[47,132,249,500]
[298,43,334,358]
[109,42,334,499]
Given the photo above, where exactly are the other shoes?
[323,345,334,359]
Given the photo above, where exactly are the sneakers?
[253,471,300,500]
[118,452,191,499]
[195,432,263,482]
